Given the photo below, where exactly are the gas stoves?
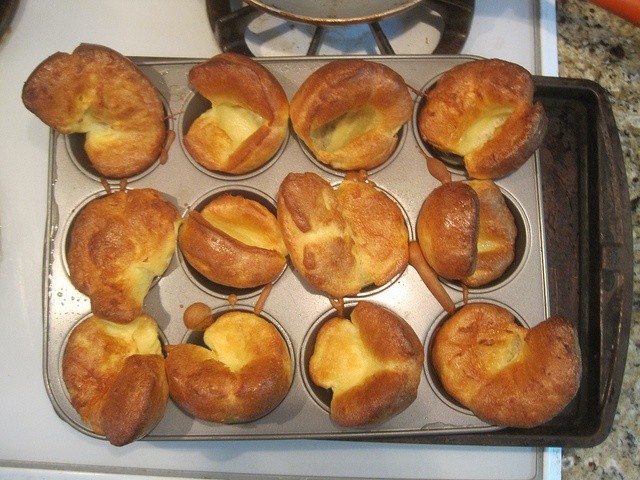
[0,1,562,480]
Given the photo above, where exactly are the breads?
[164,312,291,424]
[67,187,183,325]
[183,52,289,176]
[430,302,583,430]
[418,58,548,179]
[277,173,409,297]
[178,195,289,289]
[308,298,424,428]
[415,179,518,289]
[61,314,169,447]
[23,44,167,178]
[289,60,413,171]
